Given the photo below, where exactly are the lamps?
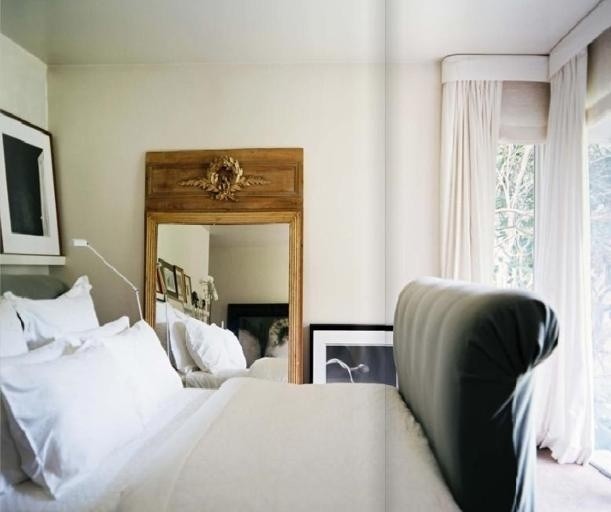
[71,237,141,320]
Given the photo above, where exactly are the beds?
[0,268,559,512]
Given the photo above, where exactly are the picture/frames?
[155,266,166,303]
[308,322,398,384]
[184,273,193,307]
[224,303,288,366]
[0,109,64,255]
[157,257,177,298]
[174,264,186,303]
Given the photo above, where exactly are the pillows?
[4,275,98,349]
[0,343,68,497]
[155,301,166,350]
[0,298,33,487]
[165,303,196,372]
[80,316,130,349]
[40,321,181,498]
[182,318,246,374]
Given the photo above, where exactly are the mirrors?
[144,210,303,384]
